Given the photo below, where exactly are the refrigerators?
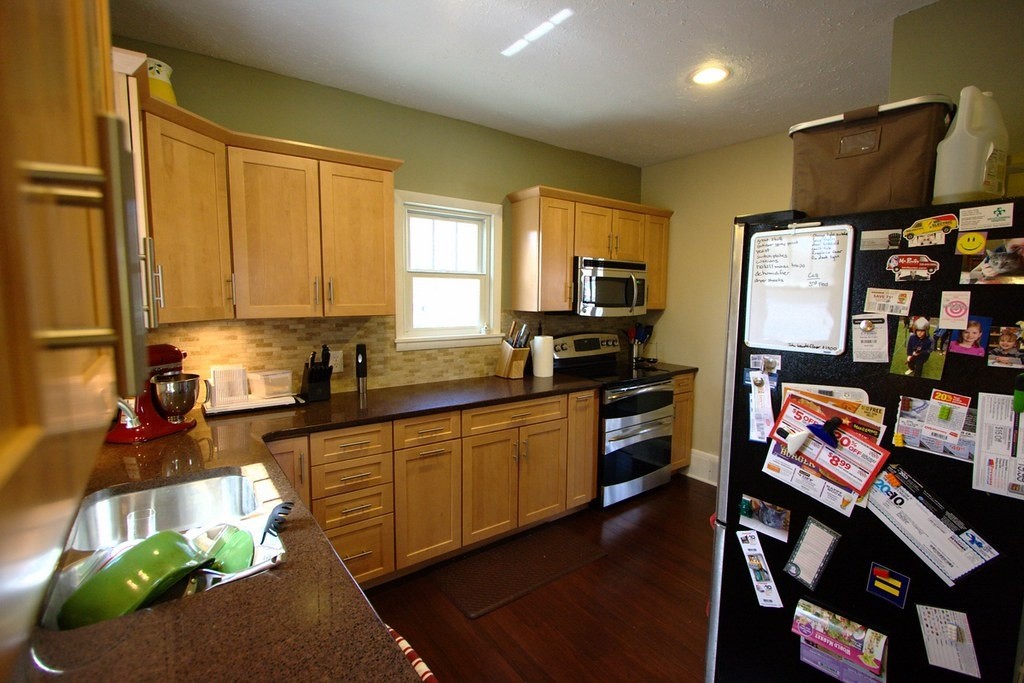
[707,196,1023,683]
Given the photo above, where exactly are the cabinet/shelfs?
[507,198,576,311]
[266,435,311,515]
[574,202,646,260]
[672,374,694,470]
[461,393,566,546]
[227,145,395,318]
[0,0,139,682]
[567,390,598,510]
[642,214,673,309]
[394,411,462,571]
[310,420,395,584]
[141,110,237,326]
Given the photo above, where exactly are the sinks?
[70,472,258,552]
[40,518,278,633]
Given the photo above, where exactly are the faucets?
[117,398,141,429]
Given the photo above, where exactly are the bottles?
[931,85,1010,205]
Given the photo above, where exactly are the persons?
[905,317,932,377]
[903,316,911,329]
[948,321,985,357]
[933,324,949,350]
[969,238,1024,279]
[988,328,1024,366]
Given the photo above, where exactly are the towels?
[383,623,439,683]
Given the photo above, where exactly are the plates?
[200,524,254,573]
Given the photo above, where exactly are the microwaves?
[572,256,649,318]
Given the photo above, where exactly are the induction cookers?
[553,332,672,389]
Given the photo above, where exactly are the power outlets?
[329,351,344,372]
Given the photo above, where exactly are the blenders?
[104,343,200,444]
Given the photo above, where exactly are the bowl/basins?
[151,375,201,422]
[56,527,216,630]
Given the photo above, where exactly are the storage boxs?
[789,94,954,217]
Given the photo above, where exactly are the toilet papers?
[529,335,554,378]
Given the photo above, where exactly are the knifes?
[507,320,531,348]
[309,343,331,369]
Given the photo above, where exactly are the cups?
[533,336,554,377]
[127,508,156,540]
[629,343,644,362]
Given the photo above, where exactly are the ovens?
[598,377,675,509]
